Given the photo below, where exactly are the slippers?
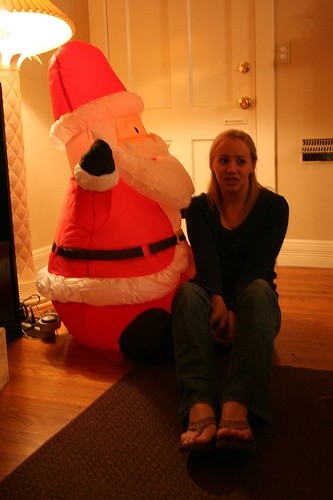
[220,418,253,444]
[182,416,217,449]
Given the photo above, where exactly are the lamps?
[0,0,77,321]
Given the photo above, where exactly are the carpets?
[1,365,332,500]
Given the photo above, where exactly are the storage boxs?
[0,327,10,391]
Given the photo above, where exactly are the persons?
[171,129,289,453]
